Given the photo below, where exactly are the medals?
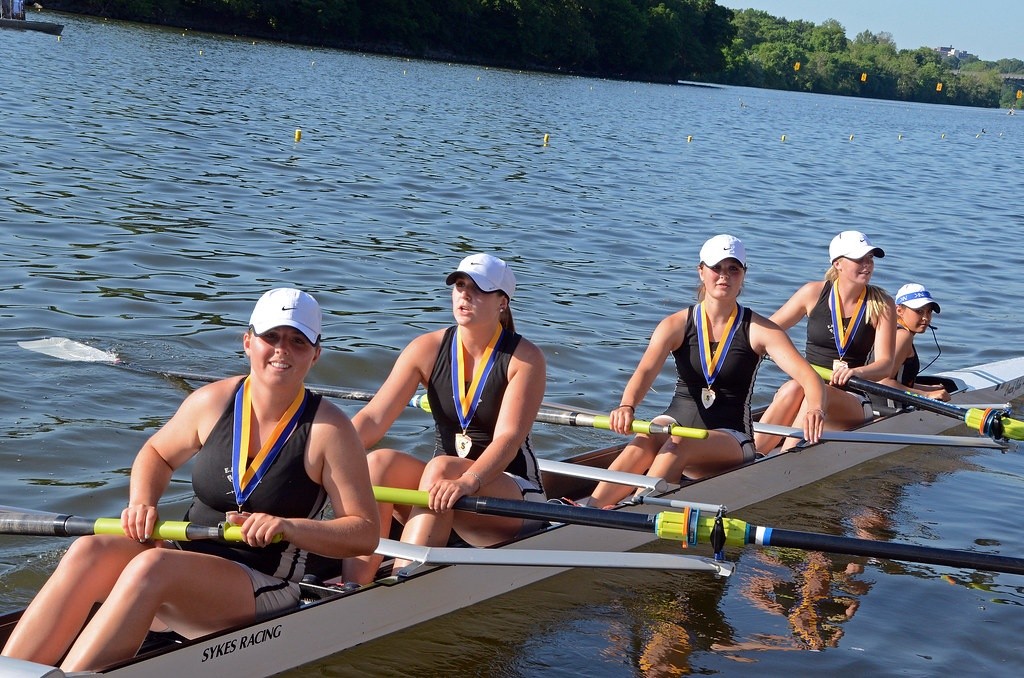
[832,360,849,373]
[701,388,716,409]
[455,433,473,458]
[225,512,251,526]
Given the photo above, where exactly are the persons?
[547,234,826,522]
[752,230,896,461]
[341,254,550,593]
[867,283,952,418]
[0,288,379,672]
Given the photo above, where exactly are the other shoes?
[547,497,583,527]
[304,577,363,603]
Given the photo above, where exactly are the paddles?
[0,505,283,544]
[762,351,1024,441]
[371,486,1024,575]
[140,366,709,439]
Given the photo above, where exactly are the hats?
[700,235,746,268]
[249,288,322,344]
[895,284,941,315]
[446,253,515,301]
[829,231,885,265]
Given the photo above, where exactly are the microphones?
[928,323,938,330]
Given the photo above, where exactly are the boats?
[0,357,1024,678]
[0,17,64,37]
